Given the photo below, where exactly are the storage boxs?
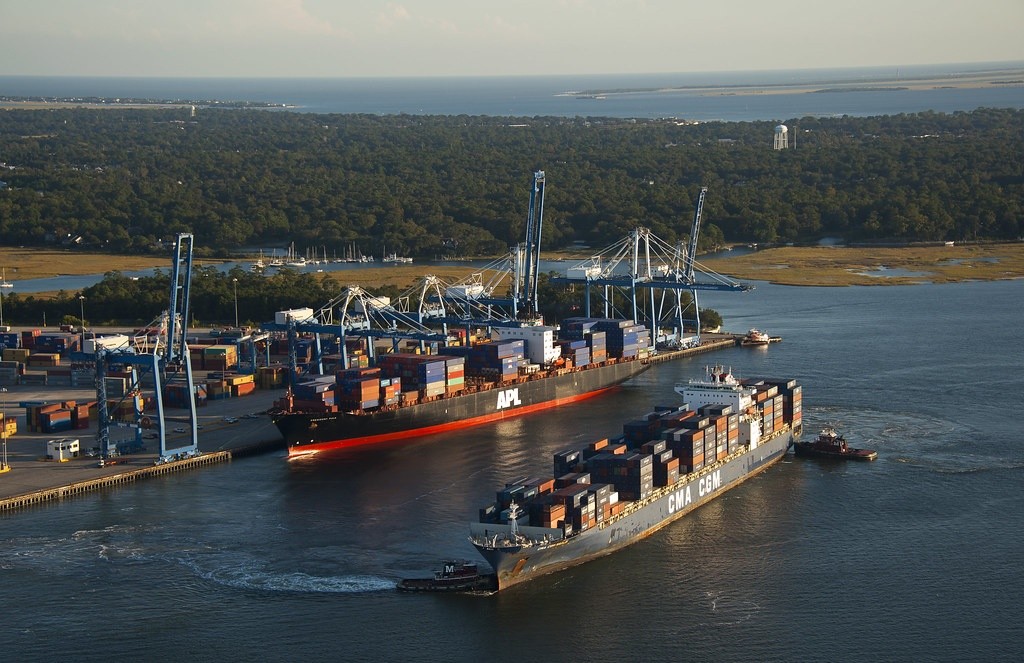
[740,378,802,437]
[0,317,738,539]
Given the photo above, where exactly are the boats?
[389,252,412,263]
[793,427,878,462]
[944,240,954,244]
[741,328,769,346]
[360,254,375,262]
[396,562,493,590]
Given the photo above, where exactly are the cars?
[197,424,203,428]
[85,452,92,456]
[175,428,186,432]
[240,413,257,417]
[224,416,238,423]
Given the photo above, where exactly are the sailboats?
[0,267,13,287]
[256,240,361,267]
[381,245,388,263]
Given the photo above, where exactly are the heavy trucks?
[97,460,116,468]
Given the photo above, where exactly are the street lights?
[1,388,7,466]
[232,277,239,328]
[79,295,85,340]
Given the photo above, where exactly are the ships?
[469,359,802,590]
[269,316,648,456]
